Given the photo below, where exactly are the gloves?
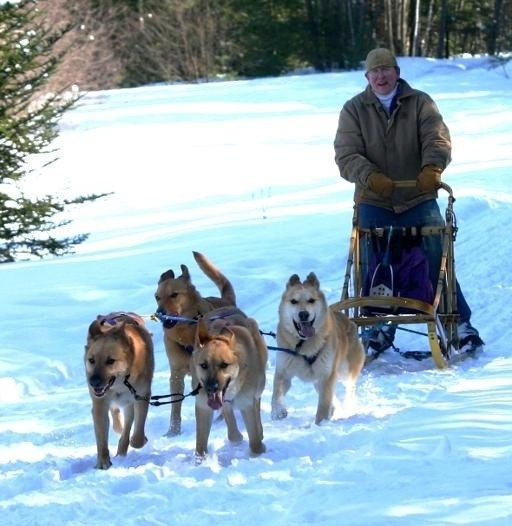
[366,170,394,199]
[415,165,443,194]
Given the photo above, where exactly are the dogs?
[191,306,269,467]
[270,271,367,426]
[153,249,237,440]
[83,311,155,470]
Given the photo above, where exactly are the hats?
[365,47,398,73]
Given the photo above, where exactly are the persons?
[334,48,484,353]
